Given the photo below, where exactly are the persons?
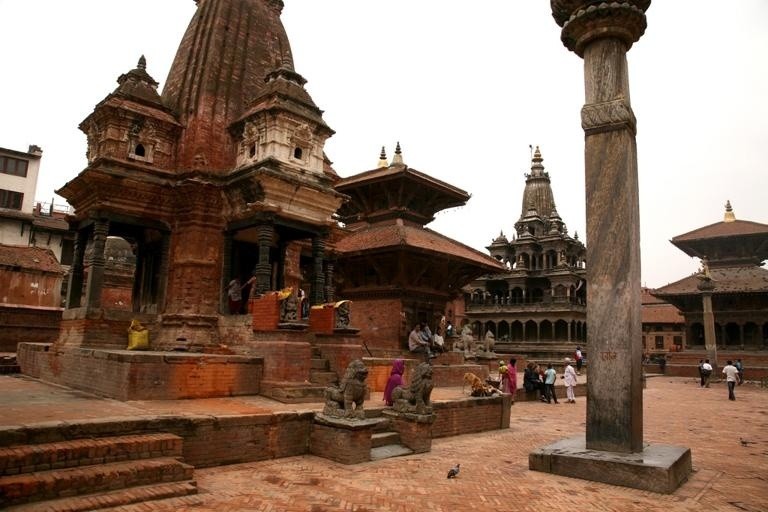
[408,319,452,367]
[226,275,242,313]
[734,358,744,386]
[235,274,257,312]
[298,287,308,318]
[496,356,559,403]
[575,345,583,374]
[561,357,578,404]
[703,359,713,388]
[697,358,705,385]
[722,360,740,401]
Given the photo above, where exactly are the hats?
[527,361,536,368]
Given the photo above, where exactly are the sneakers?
[548,401,552,404]
[554,400,560,404]
[563,400,576,403]
[539,395,548,402]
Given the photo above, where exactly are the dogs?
[462,373,485,394]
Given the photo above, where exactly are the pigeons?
[739,437,753,447]
[446,463,460,480]
